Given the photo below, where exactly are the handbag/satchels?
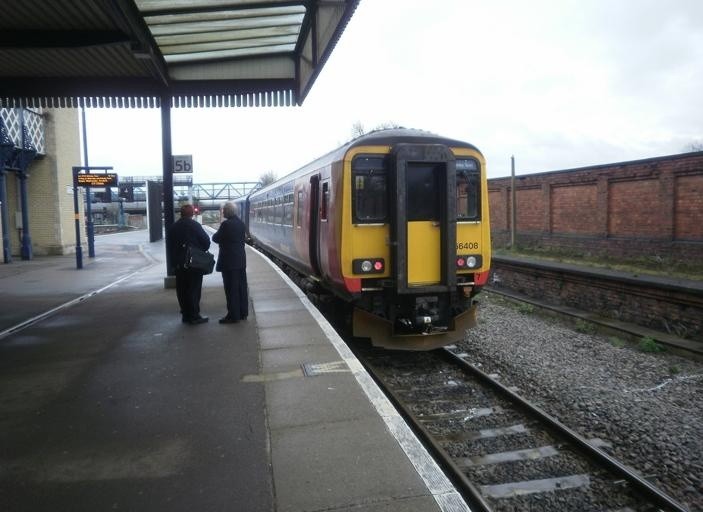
[184,243,215,275]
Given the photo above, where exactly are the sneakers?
[182,315,209,325]
[219,316,247,324]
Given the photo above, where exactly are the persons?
[211,202,248,323]
[165,204,215,324]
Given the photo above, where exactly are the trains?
[219,128,492,366]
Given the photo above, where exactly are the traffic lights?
[193,202,198,215]
